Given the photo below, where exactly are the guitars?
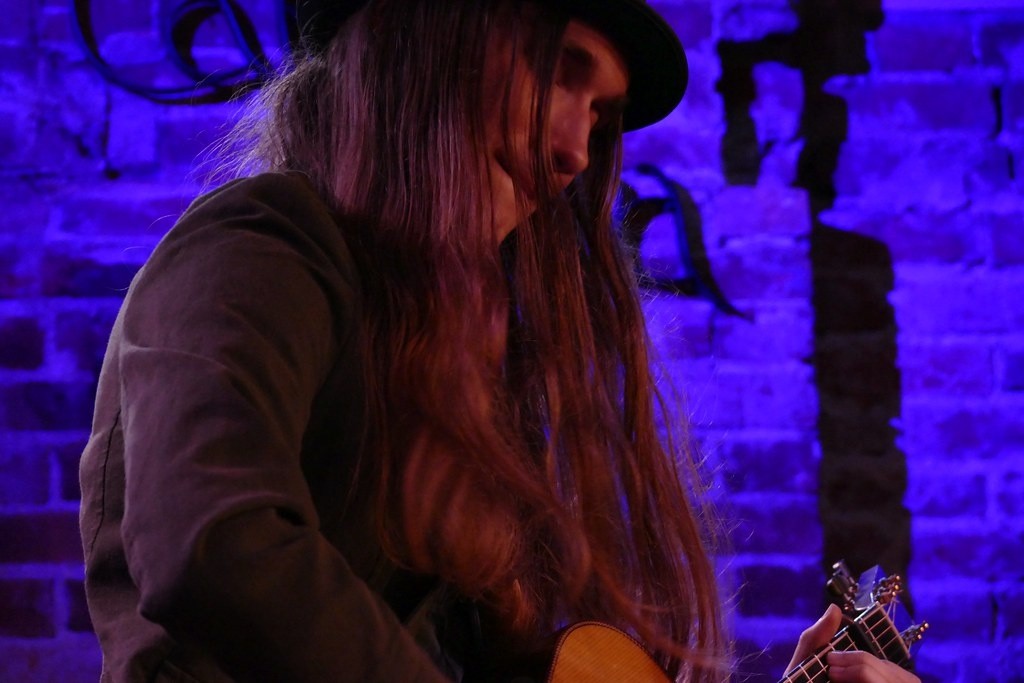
[536,558,929,682]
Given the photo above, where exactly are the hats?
[298,0,690,134]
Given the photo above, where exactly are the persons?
[78,2,929,681]
